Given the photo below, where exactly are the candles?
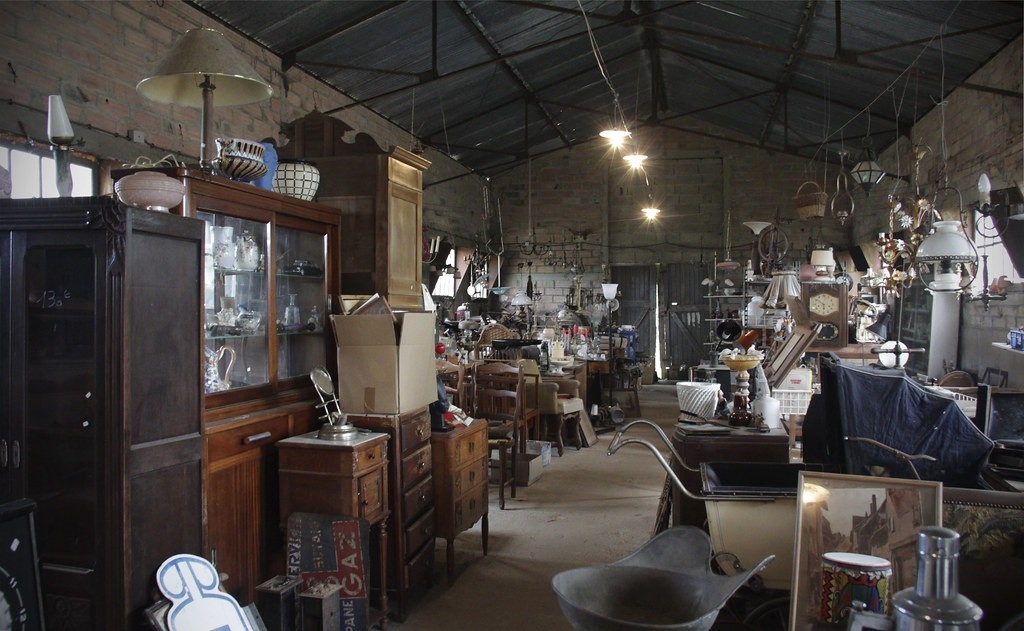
[430,238,434,253]
[435,235,440,252]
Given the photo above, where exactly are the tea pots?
[307,305,322,332]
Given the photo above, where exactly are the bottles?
[538,340,549,371]
[284,293,300,331]
[729,395,752,427]
[892,526,984,631]
[210,225,235,270]
[249,143,278,191]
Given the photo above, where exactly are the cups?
[216,307,263,332]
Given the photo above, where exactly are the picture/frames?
[789,471,944,631]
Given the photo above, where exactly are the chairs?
[435,345,580,510]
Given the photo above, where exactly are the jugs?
[234,229,260,271]
[204,345,236,394]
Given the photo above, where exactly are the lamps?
[136,28,273,174]
[598,129,661,219]
[916,29,1012,311]
[602,283,619,405]
[489,255,532,306]
[743,221,772,275]
[830,108,885,227]
[510,293,531,319]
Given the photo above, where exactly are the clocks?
[800,280,848,347]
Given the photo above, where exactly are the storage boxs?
[329,284,439,413]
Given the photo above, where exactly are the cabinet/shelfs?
[0,156,488,631]
[702,294,755,362]
